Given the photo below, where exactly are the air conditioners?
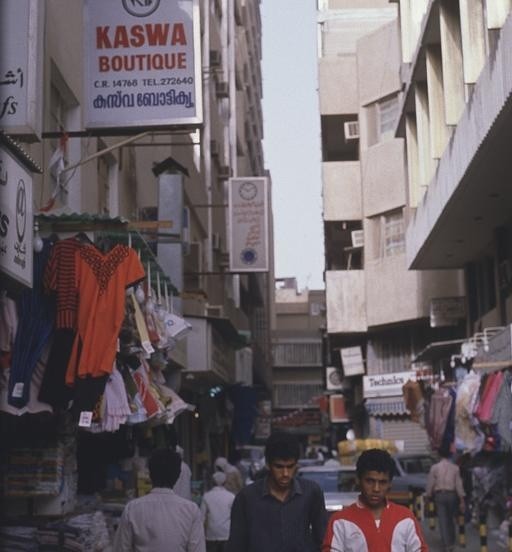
[210,49,230,97]
[326,366,352,391]
[211,139,233,180]
[343,120,360,145]
[212,232,222,253]
[350,229,365,248]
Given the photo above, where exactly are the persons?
[424,445,467,551]
[114,433,429,551]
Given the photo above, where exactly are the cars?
[228,444,437,512]
